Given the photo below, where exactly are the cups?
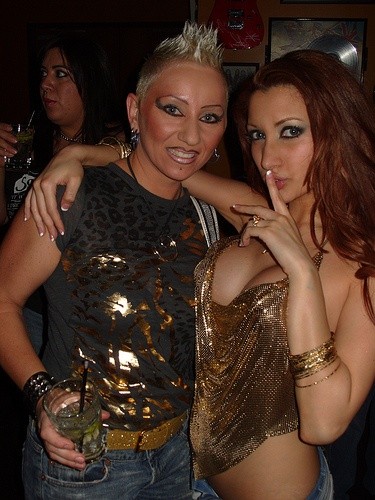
[4,128,35,168]
[43,378,105,461]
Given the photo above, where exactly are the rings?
[252,215,261,227]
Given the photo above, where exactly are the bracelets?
[22,371,57,421]
[98,135,131,160]
[289,332,340,388]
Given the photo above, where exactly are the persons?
[0,18,231,500]
[0,37,131,364]
[25,49,375,500]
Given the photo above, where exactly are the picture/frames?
[222,62,260,96]
[268,16,367,87]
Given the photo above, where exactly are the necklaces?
[262,248,270,254]
[57,129,82,141]
